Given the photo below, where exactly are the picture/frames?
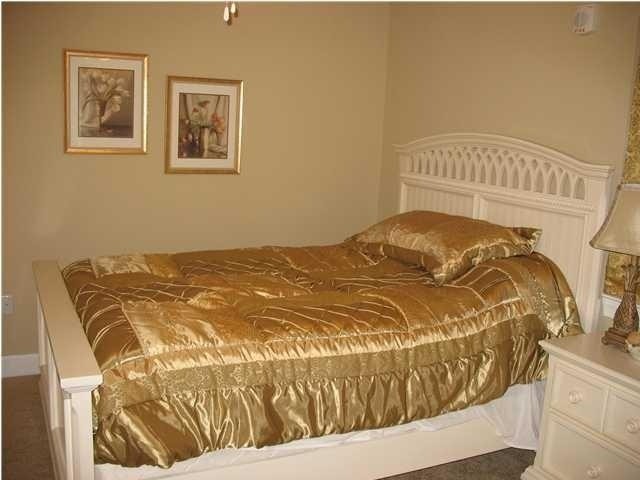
[61,48,244,176]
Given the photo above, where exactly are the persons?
[184,107,200,152]
[198,100,211,126]
[207,117,228,158]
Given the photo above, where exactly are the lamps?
[589,181,639,353]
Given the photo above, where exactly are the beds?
[29,132,617,477]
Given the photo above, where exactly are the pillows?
[338,206,547,288]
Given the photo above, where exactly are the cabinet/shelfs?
[523,332,639,480]
